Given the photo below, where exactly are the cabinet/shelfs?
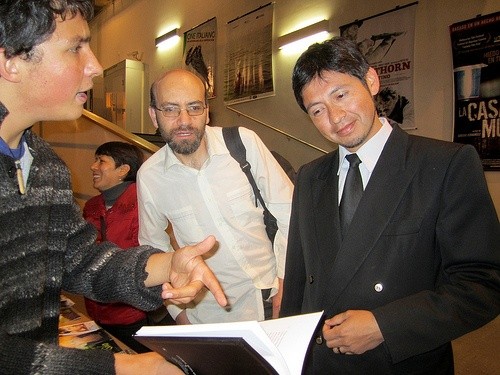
[103,59,144,134]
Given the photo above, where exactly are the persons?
[79,141,152,354]
[136,70,294,326]
[278,38,500,375]
[0,0,228,375]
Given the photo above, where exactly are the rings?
[337,347,342,353]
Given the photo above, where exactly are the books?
[134,311,324,375]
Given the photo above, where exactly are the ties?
[339,153,364,242]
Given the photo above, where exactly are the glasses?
[152,105,207,118]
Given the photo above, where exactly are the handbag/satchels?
[222,125,297,243]
